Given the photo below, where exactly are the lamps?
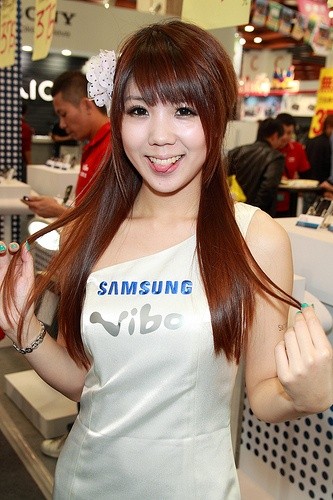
[27,219,60,254]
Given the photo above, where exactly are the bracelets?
[11,321,45,354]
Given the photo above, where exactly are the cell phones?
[23,195,31,201]
[315,199,332,218]
[63,185,73,202]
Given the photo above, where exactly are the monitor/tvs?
[20,46,90,136]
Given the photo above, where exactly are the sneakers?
[42,435,68,458]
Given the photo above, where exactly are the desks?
[27,165,80,274]
[280,186,325,217]
[231,218,333,500]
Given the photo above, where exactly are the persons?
[0,18,333,500]
[19,71,333,218]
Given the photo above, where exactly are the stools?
[0,178,39,248]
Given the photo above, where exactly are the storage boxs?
[238,89,318,119]
